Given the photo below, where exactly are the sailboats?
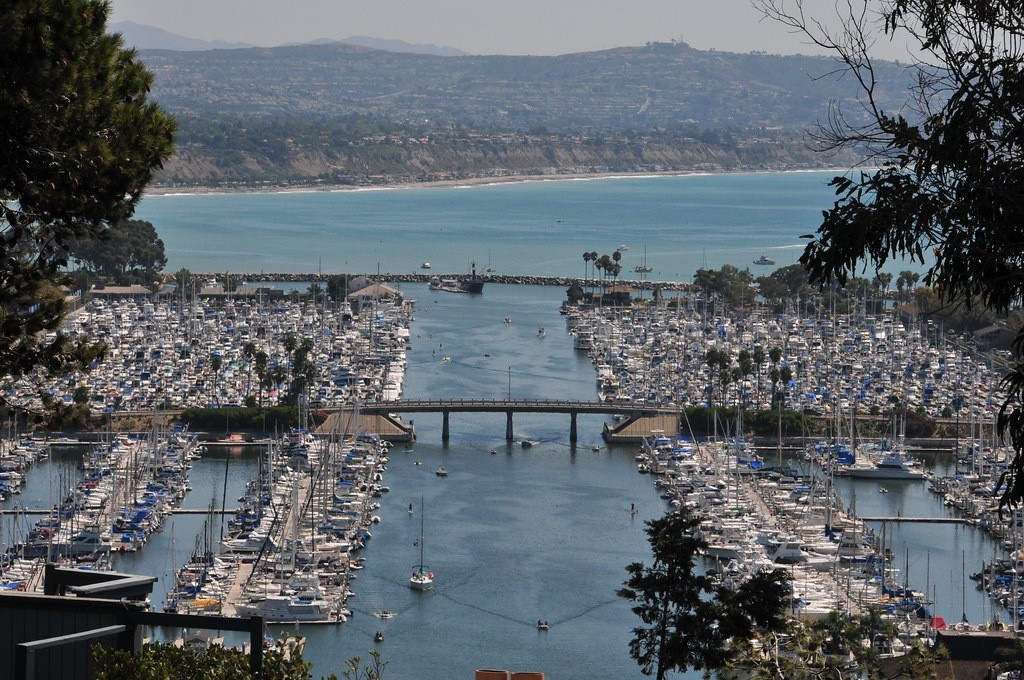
[408,494,435,593]
[0,255,419,667]
[486,248,495,273]
[460,260,484,293]
[559,241,1023,680]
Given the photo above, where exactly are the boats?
[535,624,549,630]
[421,261,432,268]
[491,450,496,454]
[443,357,450,361]
[485,353,490,357]
[435,470,448,476]
[522,441,531,448]
[427,275,467,293]
[503,320,512,324]
